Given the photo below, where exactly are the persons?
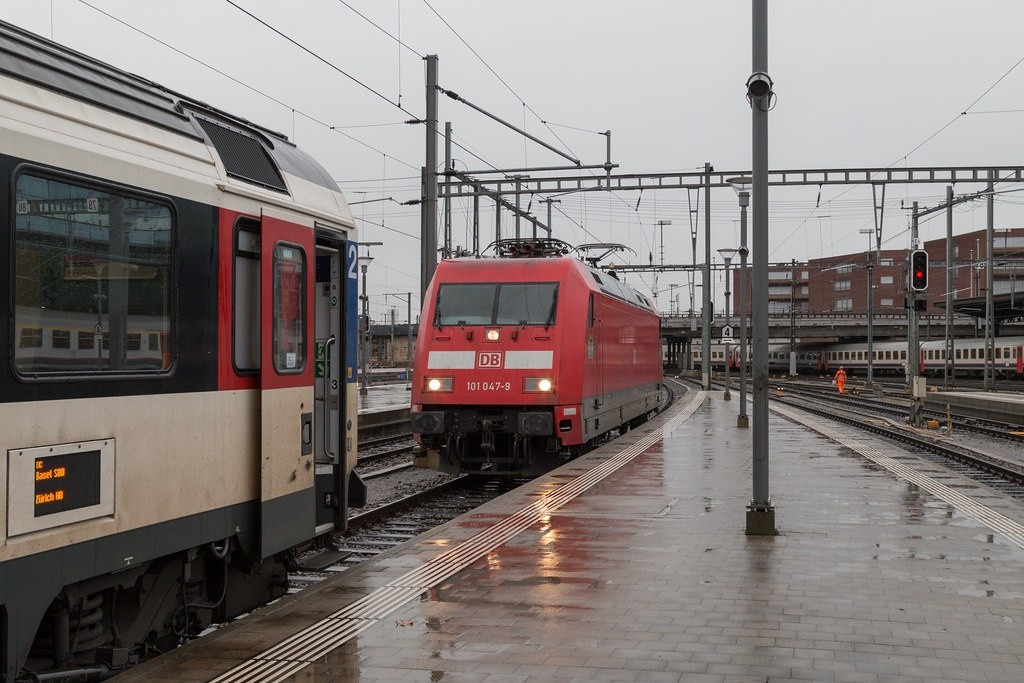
[834,366,846,394]
[39,267,168,368]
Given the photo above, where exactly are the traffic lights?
[910,249,929,291]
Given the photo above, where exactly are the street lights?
[717,248,738,400]
[358,256,374,395]
[725,176,754,428]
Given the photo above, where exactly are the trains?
[397,234,663,479]
[662,334,1024,383]
[1,12,371,683]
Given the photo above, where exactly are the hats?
[840,366,843,368]
